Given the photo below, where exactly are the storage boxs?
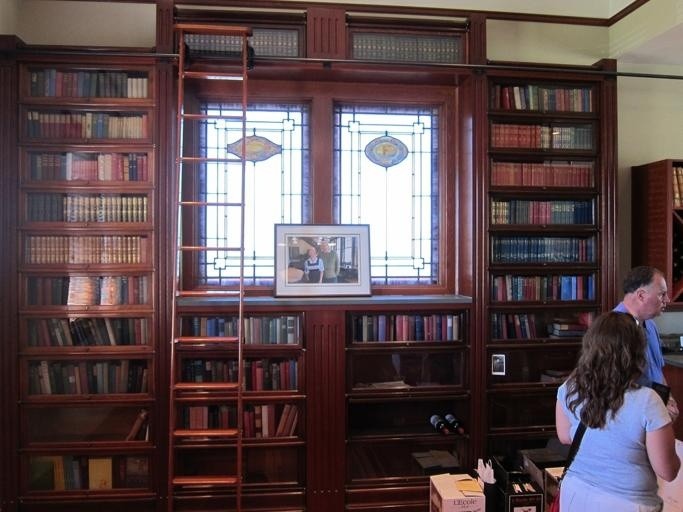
[427,471,484,511]
[491,475,543,512]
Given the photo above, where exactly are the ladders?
[167,25,255,512]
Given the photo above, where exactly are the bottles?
[444,414,464,435]
[430,415,449,434]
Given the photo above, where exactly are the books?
[677,167,683,205]
[358,313,462,341]
[488,83,597,383]
[673,167,680,208]
[181,316,300,438]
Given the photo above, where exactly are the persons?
[304,248,324,283]
[318,241,340,283]
[613,265,680,422]
[554,313,680,512]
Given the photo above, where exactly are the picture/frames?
[490,353,506,377]
[271,221,371,299]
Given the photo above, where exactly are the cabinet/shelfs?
[629,156,683,316]
[0,0,621,512]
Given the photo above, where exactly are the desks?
[657,352,682,440]
